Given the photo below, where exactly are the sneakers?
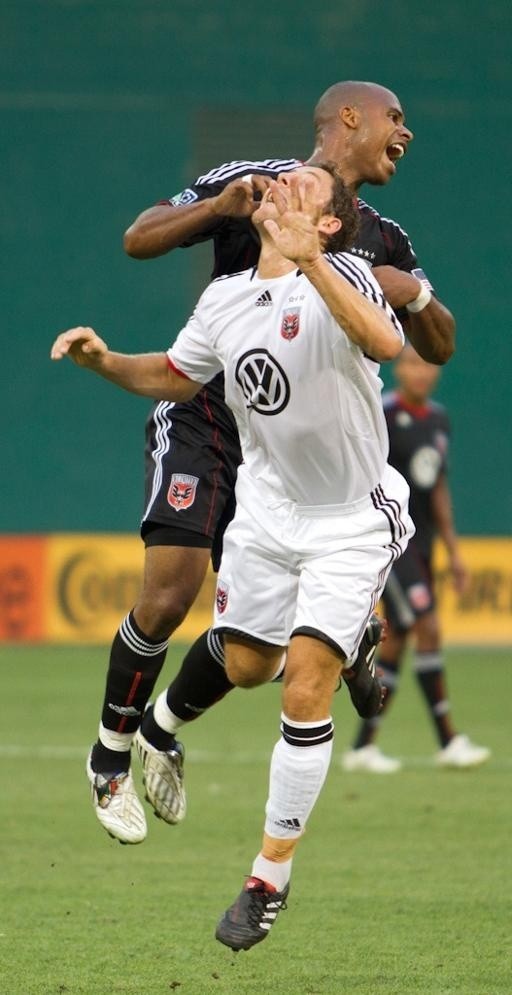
[341,744,401,775]
[342,612,387,719]
[86,756,146,842]
[215,876,289,951]
[434,734,490,767]
[133,703,187,824]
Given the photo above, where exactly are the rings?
[238,172,254,187]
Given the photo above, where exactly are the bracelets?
[403,282,435,313]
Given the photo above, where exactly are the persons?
[79,77,460,854]
[335,340,497,777]
[44,162,421,954]
